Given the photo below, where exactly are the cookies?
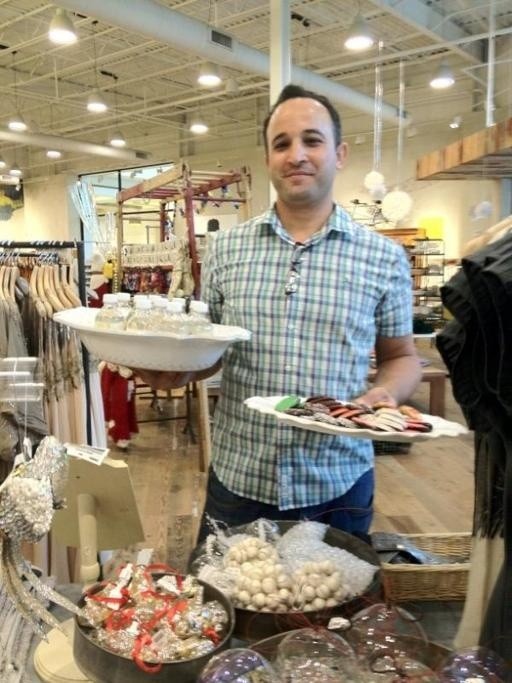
[275,393,432,433]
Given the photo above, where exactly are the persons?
[126,81,426,567]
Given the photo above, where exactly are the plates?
[243,393,469,442]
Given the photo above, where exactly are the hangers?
[0,238,100,319]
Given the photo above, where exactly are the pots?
[72,571,238,683]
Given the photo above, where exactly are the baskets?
[379,531,473,605]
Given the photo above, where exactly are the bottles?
[94,292,216,338]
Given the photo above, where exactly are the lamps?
[189,89,208,135]
[86,23,108,113]
[0,156,6,169]
[45,148,62,160]
[344,22,493,228]
[197,61,220,86]
[5,50,29,131]
[110,94,125,148]
[46,10,78,45]
[9,144,22,176]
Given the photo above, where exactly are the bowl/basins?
[52,305,253,373]
[204,625,504,683]
[188,519,381,636]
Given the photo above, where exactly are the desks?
[30,538,509,683]
[414,367,450,422]
